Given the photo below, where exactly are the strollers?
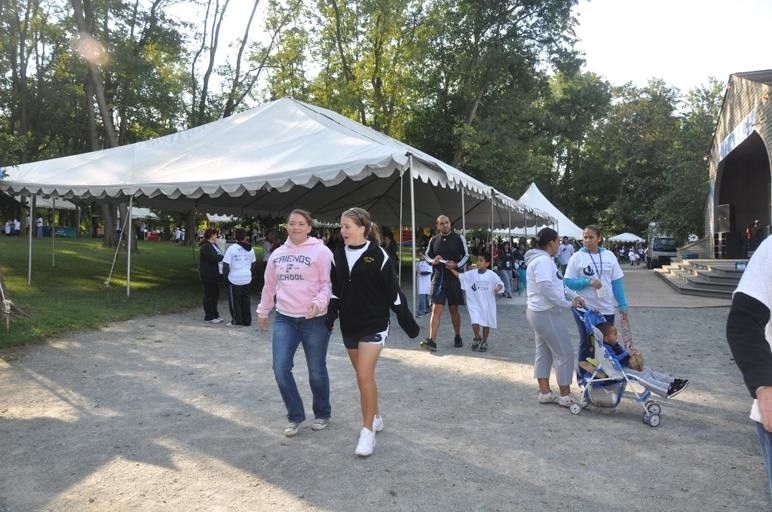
[567,299,669,430]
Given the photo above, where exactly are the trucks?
[646,234,680,271]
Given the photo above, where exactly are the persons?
[556,236,574,276]
[326,206,421,455]
[199,227,280,326]
[175,227,185,243]
[5,217,20,236]
[36,214,43,237]
[725,233,772,509]
[564,226,628,392]
[416,215,525,351]
[750,220,763,249]
[138,220,146,240]
[256,209,333,435]
[382,231,398,267]
[596,322,689,399]
[616,245,644,268]
[523,228,588,408]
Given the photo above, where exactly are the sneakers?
[454,337,463,347]
[559,394,588,409]
[284,421,305,436]
[355,415,384,456]
[312,418,329,430]
[502,293,513,298]
[666,379,688,398]
[419,338,436,351]
[417,312,431,317]
[205,316,232,327]
[472,336,488,352]
[537,390,558,403]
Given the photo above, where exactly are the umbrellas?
[608,232,644,244]
